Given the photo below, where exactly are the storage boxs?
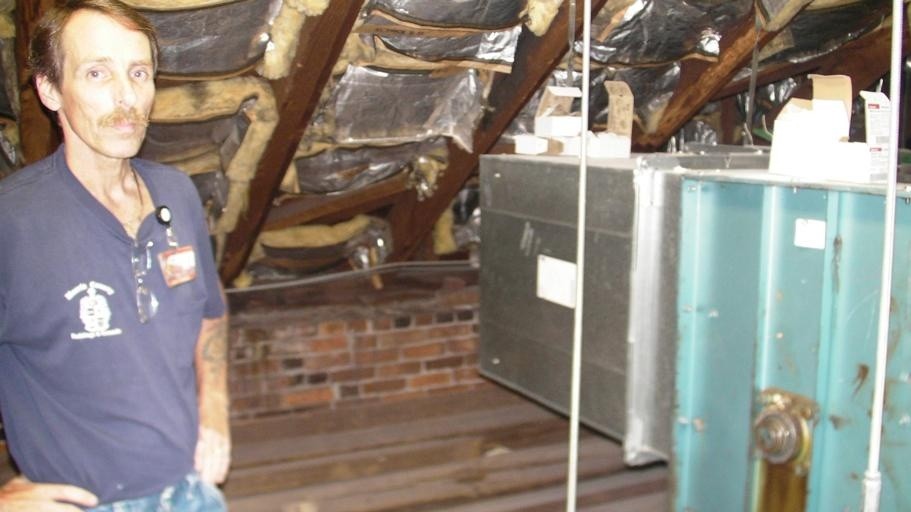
[533,84,583,150]
[767,73,853,174]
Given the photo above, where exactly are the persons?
[3,3,232,512]
